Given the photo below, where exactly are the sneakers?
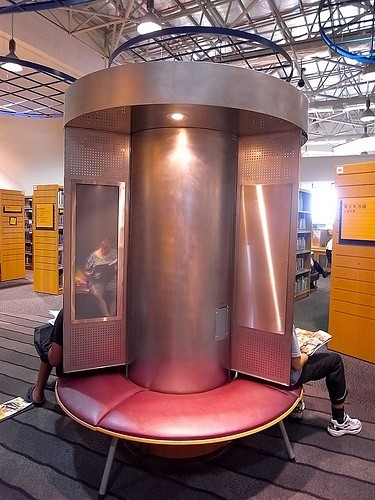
[327,412,362,436]
[294,399,305,413]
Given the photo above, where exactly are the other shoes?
[24,385,46,407]
[310,284,318,289]
[323,270,331,278]
[45,378,56,391]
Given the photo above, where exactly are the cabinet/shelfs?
[0,185,64,295]
[294,162,375,363]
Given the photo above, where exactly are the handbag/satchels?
[33,323,55,364]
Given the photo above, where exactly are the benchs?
[54,367,303,500]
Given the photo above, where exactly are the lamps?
[137,0,163,35]
[0,13,23,71]
[361,96,375,121]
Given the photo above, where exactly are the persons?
[27,308,64,407]
[83,237,117,317]
[290,324,362,437]
[326,237,332,263]
[310,258,331,289]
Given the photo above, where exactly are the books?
[298,213,311,231]
[24,198,32,263]
[299,192,304,211]
[297,235,306,250]
[295,271,308,294]
[296,328,332,356]
[48,310,59,325]
[58,190,64,287]
[0,396,34,422]
[295,257,305,273]
